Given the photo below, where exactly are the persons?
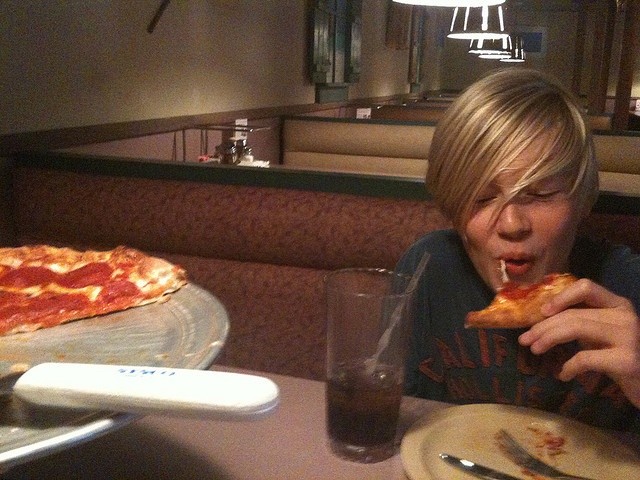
[385,66,638,434]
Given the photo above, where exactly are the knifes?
[439,450,517,479]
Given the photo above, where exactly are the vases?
[508,25,547,57]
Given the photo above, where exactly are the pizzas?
[0,242,188,337]
[466,274,577,331]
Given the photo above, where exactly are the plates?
[401,402,640,480]
[1,246,232,475]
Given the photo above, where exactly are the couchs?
[0,163,640,376]
[372,105,447,122]
[590,134,639,197]
[582,114,611,134]
[282,119,437,179]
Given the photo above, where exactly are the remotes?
[13,361,280,421]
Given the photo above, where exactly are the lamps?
[469,34,512,54]
[447,6,509,39]
[478,55,512,59]
[392,0,507,8]
[500,36,526,62]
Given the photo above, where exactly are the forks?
[493,428,595,480]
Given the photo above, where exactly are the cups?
[321,267,417,463]
